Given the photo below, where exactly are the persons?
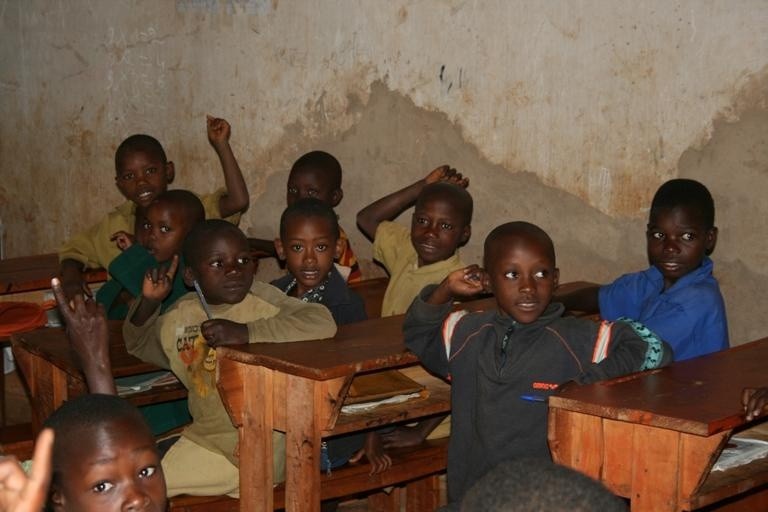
[550,179,728,366]
[741,384,768,421]
[54,115,250,302]
[356,166,474,449]
[400,221,672,500]
[268,195,392,476]
[456,456,630,511]
[96,189,206,454]
[273,151,367,285]
[0,393,169,512]
[121,220,337,499]
[49,275,116,398]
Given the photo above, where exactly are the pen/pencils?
[519,395,545,402]
[192,280,213,321]
